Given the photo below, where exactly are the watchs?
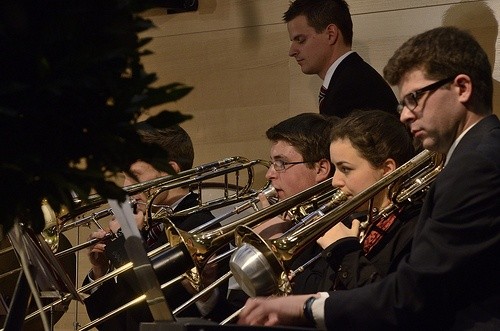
[303,296,316,328]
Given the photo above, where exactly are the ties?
[318,86,325,113]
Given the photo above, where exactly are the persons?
[80,127,231,331]
[280,0,399,124]
[293,109,417,296]
[237,25,500,331]
[182,112,336,323]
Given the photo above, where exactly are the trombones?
[0,148,447,331]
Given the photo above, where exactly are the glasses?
[268,160,308,171]
[396,78,454,113]
